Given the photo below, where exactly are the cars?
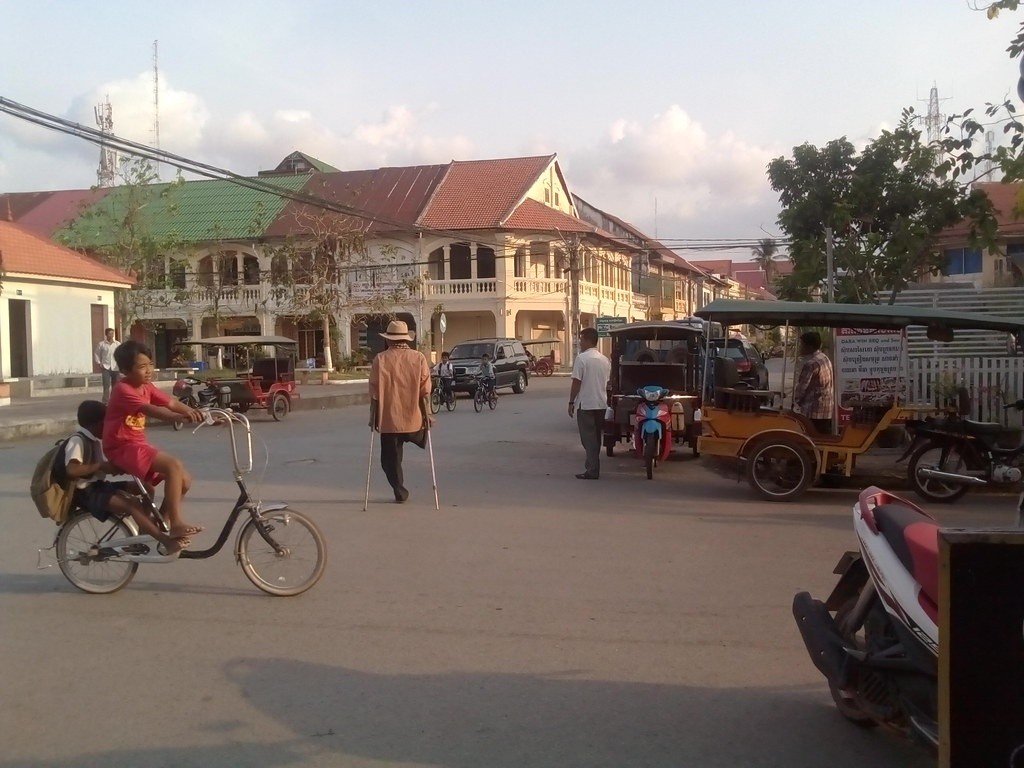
[706,338,769,390]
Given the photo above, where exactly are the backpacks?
[29,433,90,525]
[438,363,456,378]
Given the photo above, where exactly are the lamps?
[507,309,512,316]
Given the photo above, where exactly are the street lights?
[554,227,578,370]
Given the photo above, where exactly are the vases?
[875,425,905,448]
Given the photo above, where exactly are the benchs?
[239,358,294,383]
[618,361,689,395]
[538,350,560,367]
[0,373,102,402]
[351,365,373,372]
[166,367,200,379]
[294,368,332,385]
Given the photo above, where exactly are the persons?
[94,329,122,407]
[102,340,225,540]
[431,351,456,406]
[770,331,842,489]
[568,328,611,480]
[469,353,499,400]
[53,400,191,555]
[368,321,436,504]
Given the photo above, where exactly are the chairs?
[713,356,786,412]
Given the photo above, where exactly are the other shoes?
[493,393,498,399]
[450,397,454,402]
[575,473,599,480]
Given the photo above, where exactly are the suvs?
[431,338,531,398]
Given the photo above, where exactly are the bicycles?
[430,375,457,414]
[36,408,328,598]
[471,375,498,413]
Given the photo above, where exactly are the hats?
[378,321,415,341]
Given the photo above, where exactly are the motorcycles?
[791,486,939,751]
[602,321,706,480]
[896,399,1024,503]
[172,377,227,431]
